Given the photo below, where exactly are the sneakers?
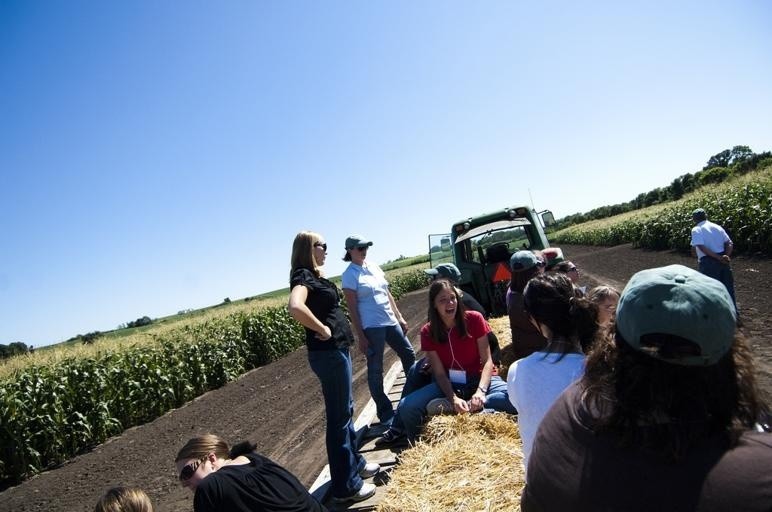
[374,428,403,449]
[331,482,377,505]
[380,411,396,426]
[360,463,380,479]
[396,431,420,447]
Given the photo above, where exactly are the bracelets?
[722,254,731,262]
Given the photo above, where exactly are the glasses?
[603,304,618,309]
[315,243,327,251]
[567,266,577,274]
[354,245,369,252]
[178,455,206,481]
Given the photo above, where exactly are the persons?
[176,432,328,511]
[93,484,153,512]
[517,262,772,510]
[687,206,744,327]
[340,234,419,432]
[286,228,380,505]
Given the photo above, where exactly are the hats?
[423,263,462,281]
[543,247,565,266]
[614,263,738,367]
[692,208,706,218]
[345,235,373,249]
[510,250,543,273]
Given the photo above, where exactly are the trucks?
[425,206,565,316]
